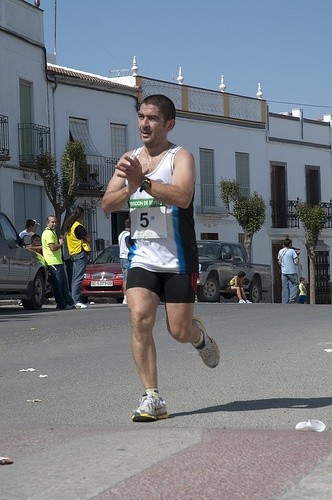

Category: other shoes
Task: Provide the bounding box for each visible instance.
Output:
[56,304,75,310]
[75,302,87,309]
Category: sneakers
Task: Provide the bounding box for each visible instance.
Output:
[239,299,252,304]
[131,394,169,422]
[191,316,220,368]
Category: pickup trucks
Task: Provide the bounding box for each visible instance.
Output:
[196,240,272,303]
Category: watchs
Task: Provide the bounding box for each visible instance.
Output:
[140,177,151,193]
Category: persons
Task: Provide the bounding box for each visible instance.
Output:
[102,94,221,421]
[118,219,132,305]
[17,206,91,309]
[224,271,252,304]
[298,277,309,304]
[278,239,299,304]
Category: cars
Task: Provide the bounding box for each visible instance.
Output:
[78,244,125,304]
[0,211,48,310]
